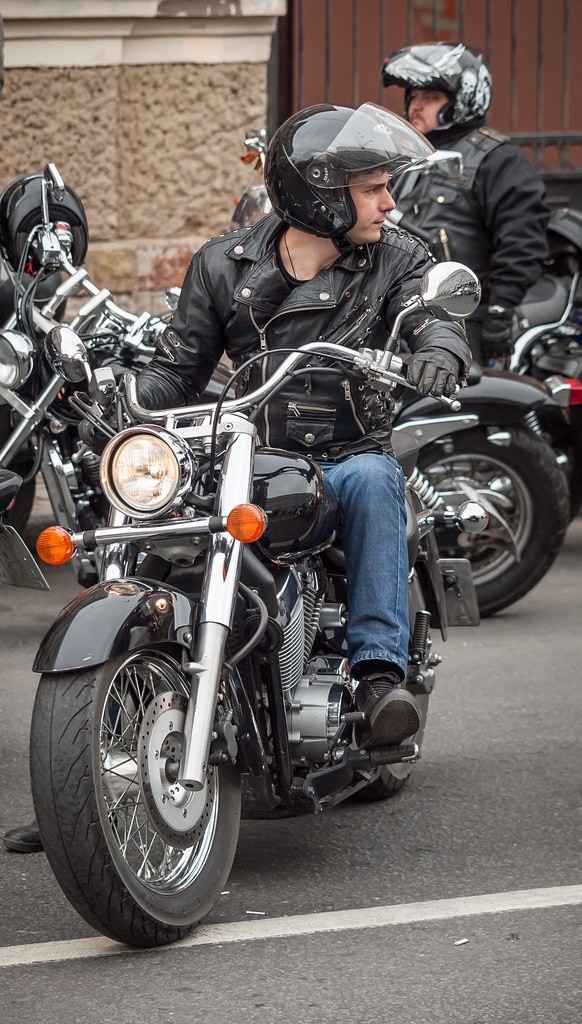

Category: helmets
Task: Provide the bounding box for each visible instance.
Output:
[264,104,413,239]
[0,171,88,273]
[380,41,493,145]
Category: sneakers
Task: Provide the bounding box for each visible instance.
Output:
[355,677,421,751]
[3,808,118,852]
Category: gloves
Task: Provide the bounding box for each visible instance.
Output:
[480,303,515,367]
[406,346,461,396]
[79,401,131,445]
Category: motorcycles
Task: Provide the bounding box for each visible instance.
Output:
[0,126,582,948]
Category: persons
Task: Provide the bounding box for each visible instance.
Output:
[2,102,474,853]
[381,40,553,358]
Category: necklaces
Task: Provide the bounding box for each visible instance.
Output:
[283,229,334,281]
